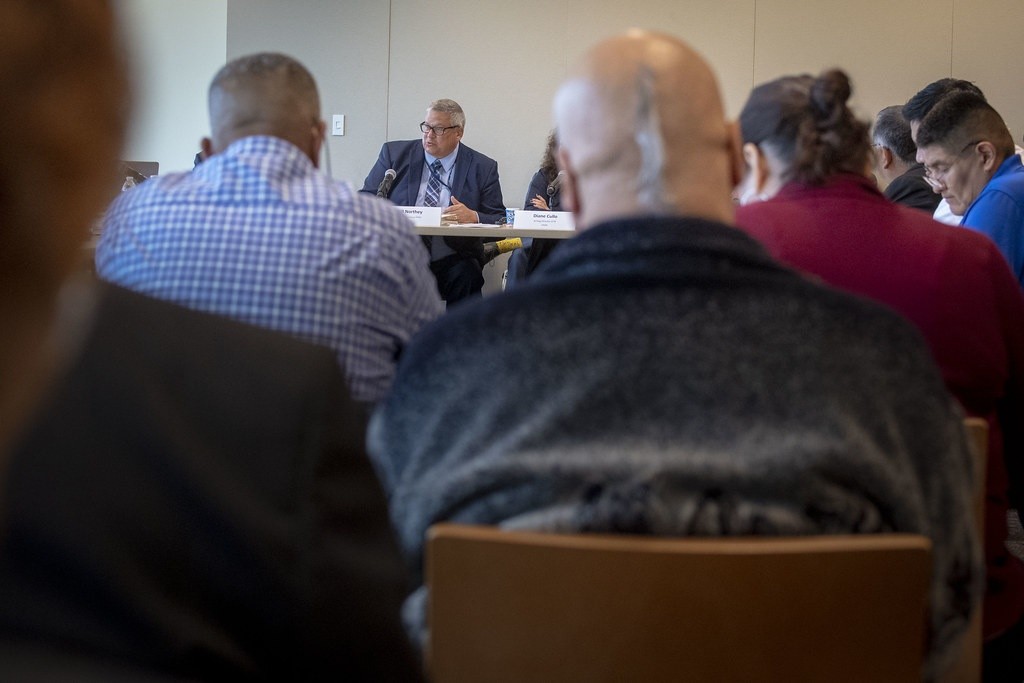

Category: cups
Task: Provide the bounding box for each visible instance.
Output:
[506,207,519,225]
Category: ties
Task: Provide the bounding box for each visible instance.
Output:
[420,160,441,266]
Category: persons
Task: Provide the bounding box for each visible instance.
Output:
[93,51,446,416]
[506,129,565,289]
[1,2,435,682]
[356,98,507,312]
[914,90,1024,285]
[902,75,1024,227]
[367,28,982,660]
[870,104,943,217]
[729,65,1024,681]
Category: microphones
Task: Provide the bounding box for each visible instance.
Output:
[118,161,148,185]
[547,169,568,196]
[376,169,396,198]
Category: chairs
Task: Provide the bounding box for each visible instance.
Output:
[425,524,931,683]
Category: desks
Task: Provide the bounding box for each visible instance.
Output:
[394,205,578,240]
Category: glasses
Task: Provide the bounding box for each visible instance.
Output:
[923,141,977,187]
[420,121,460,136]
